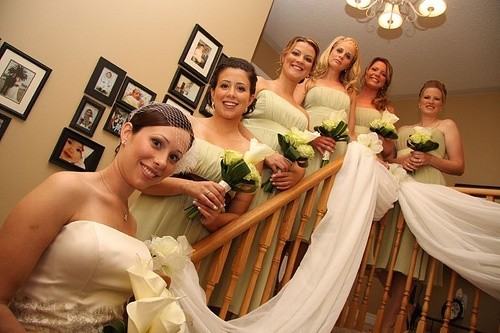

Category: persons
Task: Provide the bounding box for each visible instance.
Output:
[355,57,398,265]
[1,69,22,102]
[198,36,310,317]
[0,102,196,333]
[97,72,112,93]
[191,44,209,67]
[181,81,193,96]
[130,57,263,276]
[124,87,147,109]
[287,36,362,280]
[110,114,125,134]
[79,107,93,128]
[372,81,465,333]
[60,138,94,170]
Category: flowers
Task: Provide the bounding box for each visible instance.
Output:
[314,119,351,168]
[184,137,274,224]
[264,126,320,193]
[369,107,399,140]
[406,126,440,175]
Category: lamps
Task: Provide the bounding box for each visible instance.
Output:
[346,0,447,30]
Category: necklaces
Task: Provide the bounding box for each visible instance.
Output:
[100,171,131,221]
[433,119,439,129]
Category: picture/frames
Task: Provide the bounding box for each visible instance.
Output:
[0,41,53,121]
[209,53,229,85]
[168,66,206,110]
[199,86,215,117]
[178,23,223,84]
[84,56,127,107]
[48,127,105,172]
[162,94,194,119]
[103,102,132,138]
[0,113,12,140]
[116,76,157,111]
[69,95,106,138]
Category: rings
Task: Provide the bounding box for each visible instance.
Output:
[407,163,409,166]
[418,159,420,162]
